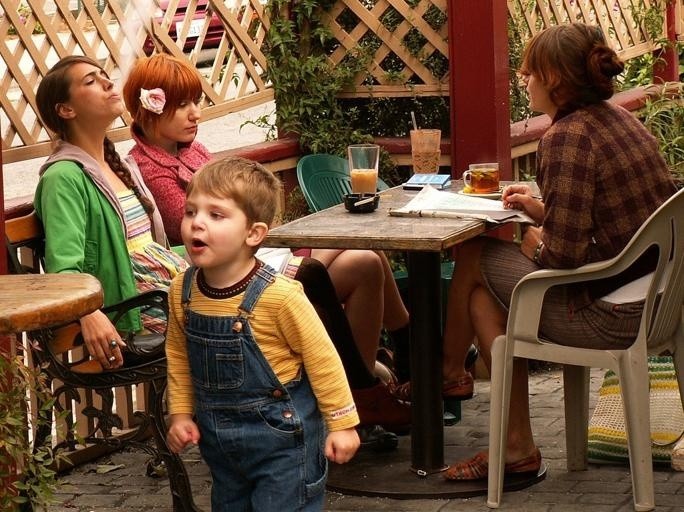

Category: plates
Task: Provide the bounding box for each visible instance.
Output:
[457,186,505,199]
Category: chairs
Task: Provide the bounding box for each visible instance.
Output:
[0,209,202,512]
[295,153,684,511]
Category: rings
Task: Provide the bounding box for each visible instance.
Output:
[108,356,116,361]
[108,338,117,347]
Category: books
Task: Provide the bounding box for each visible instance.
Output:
[386,184,538,227]
[401,172,452,191]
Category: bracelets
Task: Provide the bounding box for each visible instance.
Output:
[533,241,545,263]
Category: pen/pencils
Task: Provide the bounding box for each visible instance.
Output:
[532,196,543,199]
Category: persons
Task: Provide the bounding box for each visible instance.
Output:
[162,154,363,511]
[121,56,481,453]
[33,54,414,438]
[387,23,679,484]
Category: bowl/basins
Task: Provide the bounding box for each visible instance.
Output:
[345,194,378,214]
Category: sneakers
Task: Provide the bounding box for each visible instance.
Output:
[354,316,479,451]
[445,447,541,482]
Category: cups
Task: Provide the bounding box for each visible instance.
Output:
[348,143,380,194]
[409,129,442,176]
[462,162,501,193]
